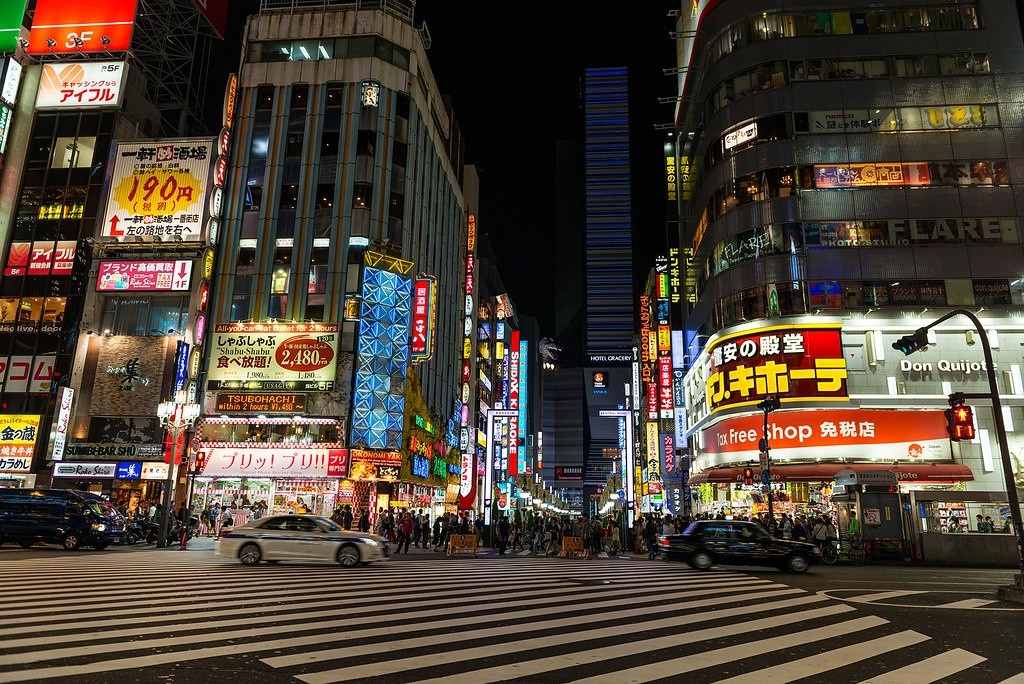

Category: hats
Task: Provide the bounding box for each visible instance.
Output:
[344,505,351,510]
[986,516,991,521]
[1007,516,1012,519]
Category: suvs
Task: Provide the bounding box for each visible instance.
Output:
[0,487,131,552]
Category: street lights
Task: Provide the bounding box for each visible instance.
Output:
[155,390,201,550]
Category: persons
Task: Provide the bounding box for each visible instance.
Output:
[357,508,370,532]
[496,512,621,561]
[949,516,960,533]
[975,514,996,533]
[169,500,233,542]
[631,514,726,561]
[374,507,429,555]
[248,505,264,522]
[732,512,837,563]
[118,499,162,521]
[849,510,860,536]
[1004,516,1013,534]
[431,511,484,552]
[329,504,353,531]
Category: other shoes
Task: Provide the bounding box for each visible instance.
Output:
[434,549,439,552]
[394,551,400,554]
[416,545,421,548]
[423,547,429,549]
[437,545,441,547]
[404,552,407,554]
[443,550,447,552]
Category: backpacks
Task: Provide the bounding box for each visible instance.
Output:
[415,517,421,529]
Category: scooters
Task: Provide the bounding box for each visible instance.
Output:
[123,509,200,547]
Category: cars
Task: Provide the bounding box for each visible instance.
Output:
[657,519,822,575]
[216,513,396,568]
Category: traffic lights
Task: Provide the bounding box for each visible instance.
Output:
[757,399,777,414]
[744,468,753,485]
[892,326,931,356]
[951,405,976,442]
[194,451,206,468]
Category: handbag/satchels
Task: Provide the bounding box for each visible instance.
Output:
[228,518,233,526]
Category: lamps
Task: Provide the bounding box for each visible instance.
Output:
[74,38,90,58]
[87,330,101,335]
[104,329,116,336]
[135,236,144,242]
[151,328,165,337]
[972,306,989,317]
[815,307,826,318]
[172,235,183,242]
[100,35,116,57]
[168,329,181,336]
[19,38,39,61]
[865,306,878,319]
[110,236,119,244]
[674,315,752,376]
[86,238,96,243]
[915,307,935,319]
[47,37,64,62]
[153,234,162,243]
[228,317,324,327]
[219,413,305,420]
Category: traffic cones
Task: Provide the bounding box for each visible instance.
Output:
[177,532,190,551]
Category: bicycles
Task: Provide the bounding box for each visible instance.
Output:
[820,533,867,566]
